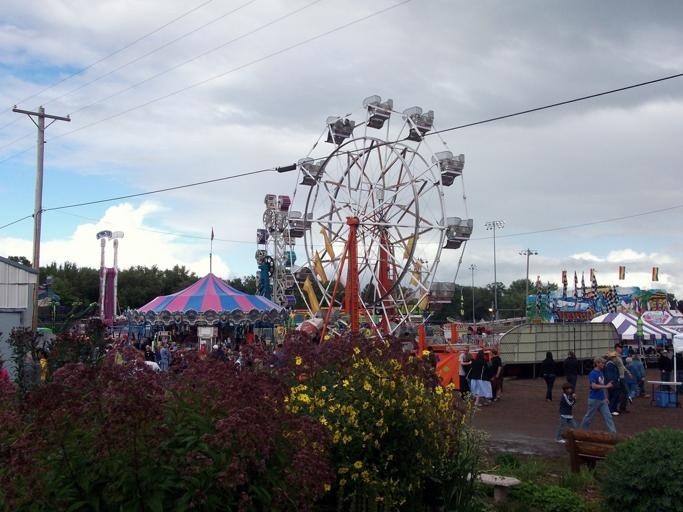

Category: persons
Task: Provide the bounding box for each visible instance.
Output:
[458,342,683,444]
[0,334,283,386]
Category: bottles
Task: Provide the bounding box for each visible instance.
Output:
[636,316,643,336]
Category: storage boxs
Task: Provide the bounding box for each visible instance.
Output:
[653,387,677,408]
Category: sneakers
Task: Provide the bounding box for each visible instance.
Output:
[612,411,619,416]
[555,438,565,443]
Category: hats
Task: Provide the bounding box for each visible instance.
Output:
[594,358,606,363]
[608,352,617,357]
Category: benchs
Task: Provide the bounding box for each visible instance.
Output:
[558,425,636,479]
